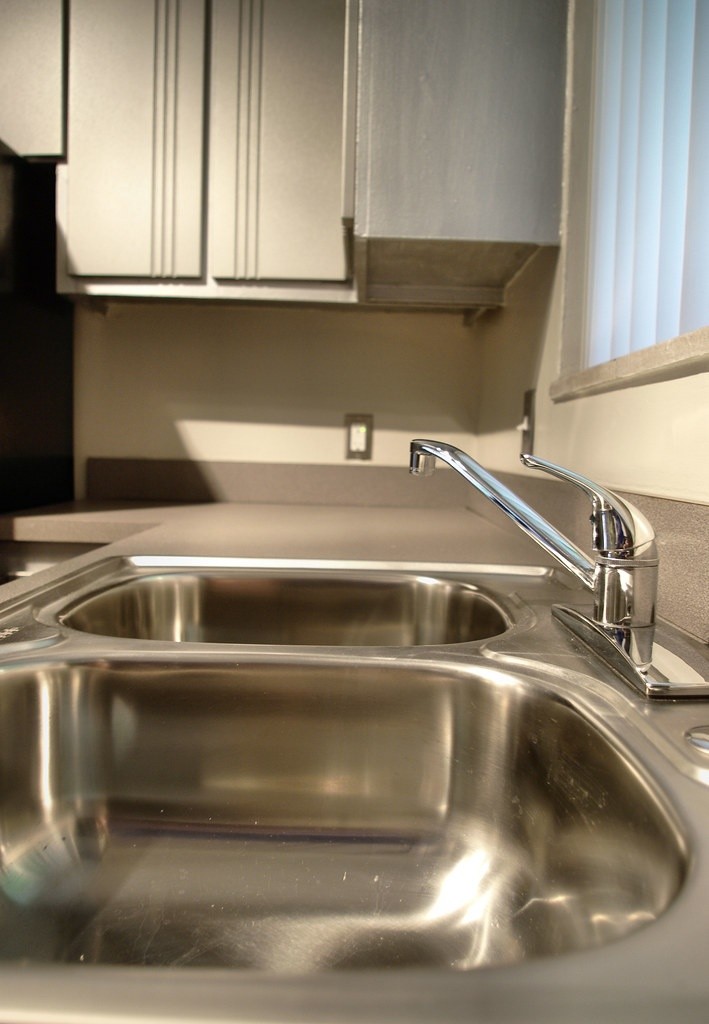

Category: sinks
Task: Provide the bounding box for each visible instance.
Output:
[0,653,709,1022]
[20,552,603,650]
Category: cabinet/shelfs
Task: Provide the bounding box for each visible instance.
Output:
[0,0,565,515]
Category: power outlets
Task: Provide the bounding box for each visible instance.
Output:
[344,413,375,458]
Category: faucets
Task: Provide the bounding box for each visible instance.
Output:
[406,436,654,627]
